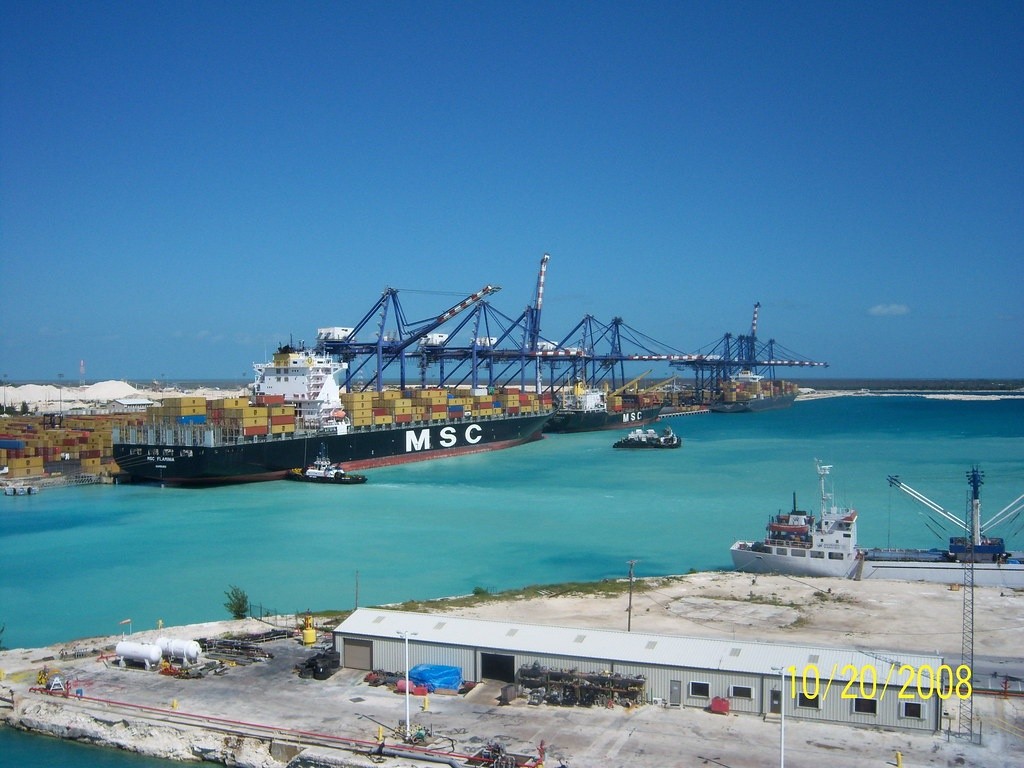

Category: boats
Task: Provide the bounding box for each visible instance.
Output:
[542,377,667,434]
[287,441,368,485]
[710,371,800,413]
[612,428,682,450]
[728,456,1024,586]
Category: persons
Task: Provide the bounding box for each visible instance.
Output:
[306,608,312,615]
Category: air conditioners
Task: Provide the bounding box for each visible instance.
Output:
[652,698,662,705]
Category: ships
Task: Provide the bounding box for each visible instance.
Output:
[112,332,565,484]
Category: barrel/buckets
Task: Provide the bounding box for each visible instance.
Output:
[76,689,82,698]
